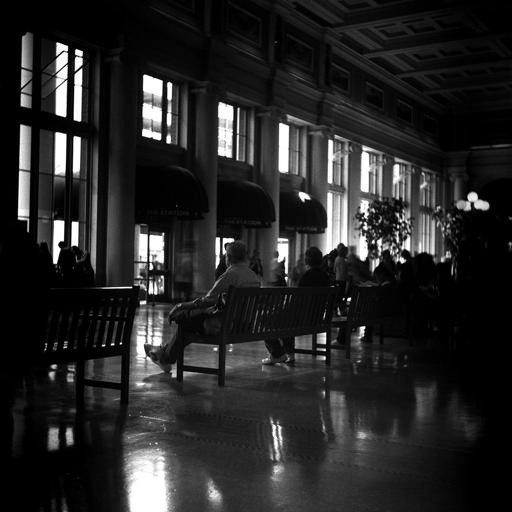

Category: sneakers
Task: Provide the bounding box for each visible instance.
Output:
[261,354,288,365]
[285,353,296,363]
[149,345,176,365]
[144,343,173,373]
[331,339,347,347]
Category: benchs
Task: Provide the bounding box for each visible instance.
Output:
[312,286,414,359]
[177,285,340,386]
[35,285,140,405]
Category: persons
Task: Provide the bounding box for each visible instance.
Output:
[143,241,263,373]
[2,225,94,390]
[262,242,369,365]
[361,225,510,359]
